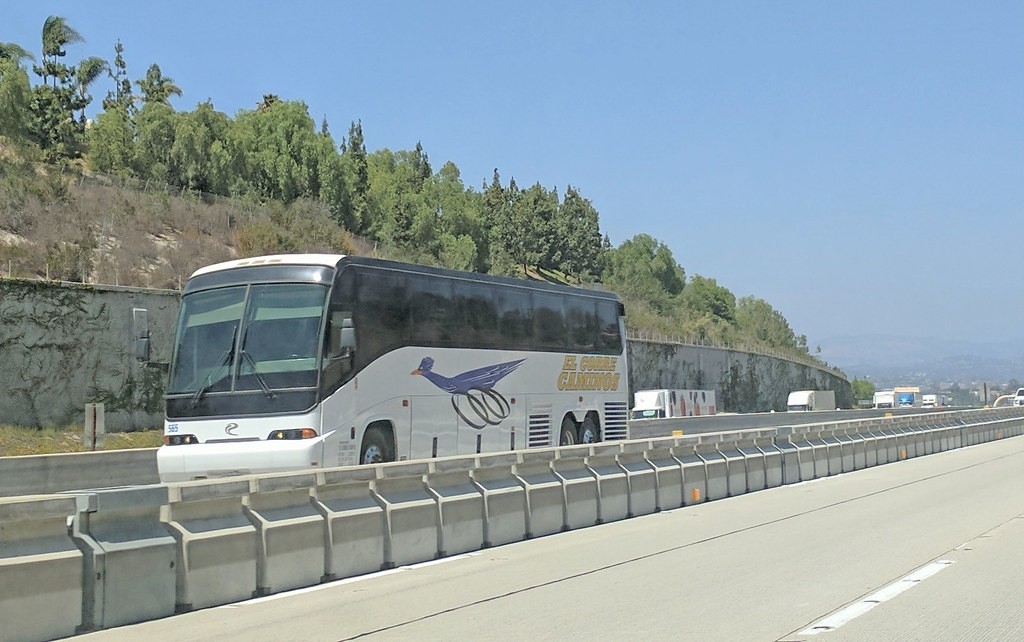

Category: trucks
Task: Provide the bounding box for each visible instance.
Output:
[787,389,836,411]
[632,390,717,419]
[873,387,942,410]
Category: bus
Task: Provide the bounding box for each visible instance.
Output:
[135,253,632,487]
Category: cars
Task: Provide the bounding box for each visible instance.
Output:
[1012,388,1023,407]
